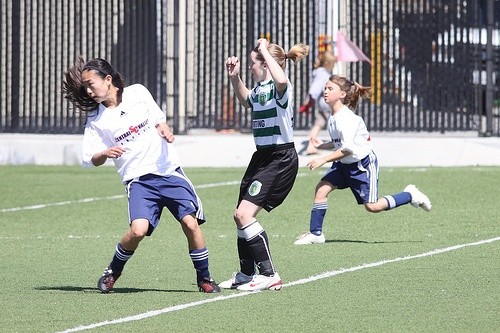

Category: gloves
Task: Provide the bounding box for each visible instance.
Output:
[299,98,315,114]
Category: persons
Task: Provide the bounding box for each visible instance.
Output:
[217,38,309,291]
[299,52,337,154]
[60,56,221,293]
[294,75,431,245]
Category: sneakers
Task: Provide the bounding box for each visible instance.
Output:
[218,269,283,291]
[97,267,121,293]
[403,184,432,211]
[197,277,222,293]
[293,231,325,245]
